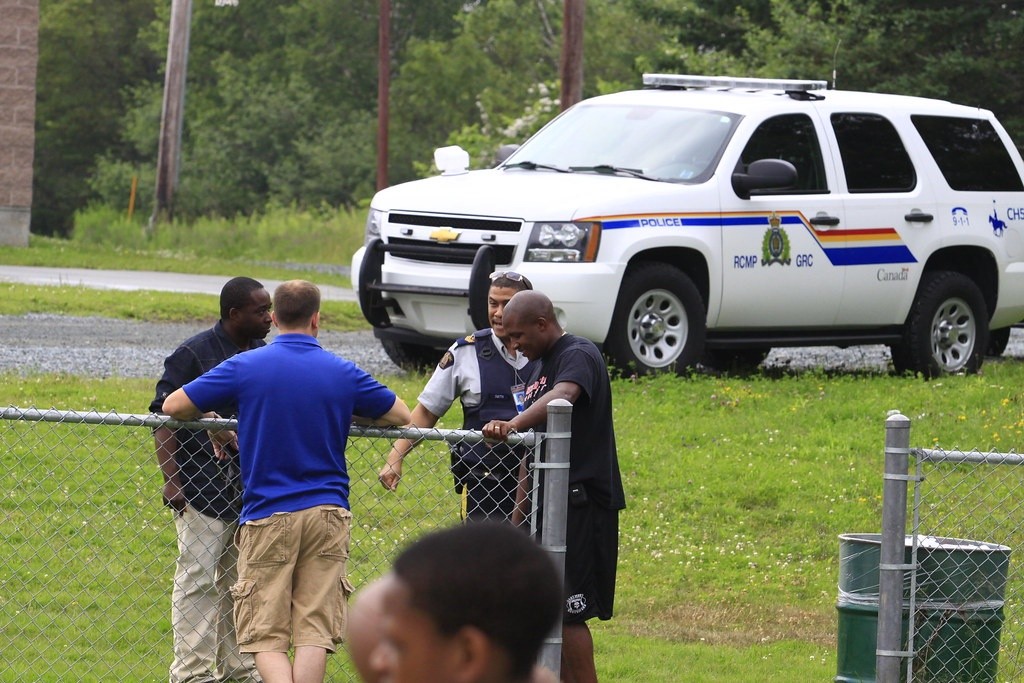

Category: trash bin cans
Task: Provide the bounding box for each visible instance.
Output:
[826,531,1012,683]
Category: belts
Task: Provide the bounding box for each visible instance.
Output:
[473,469,518,483]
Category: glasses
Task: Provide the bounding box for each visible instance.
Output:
[489,271,530,289]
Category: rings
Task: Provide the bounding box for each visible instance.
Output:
[494,426,500,429]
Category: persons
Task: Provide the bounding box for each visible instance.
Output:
[160,278,412,682]
[481,288,628,683]
[146,276,277,682]
[376,271,545,523]
[517,394,524,404]
[367,516,565,682]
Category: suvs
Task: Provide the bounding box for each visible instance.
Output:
[349,71,1024,384]
